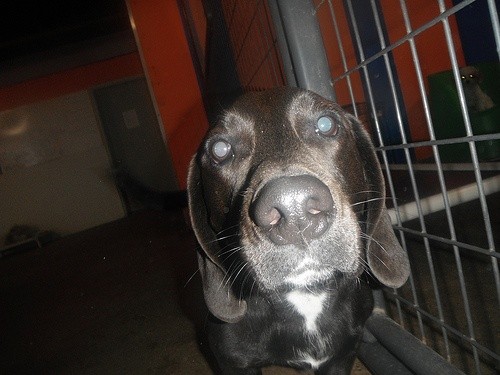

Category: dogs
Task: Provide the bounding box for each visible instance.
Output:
[120,85,411,375]
[451,66,494,114]
